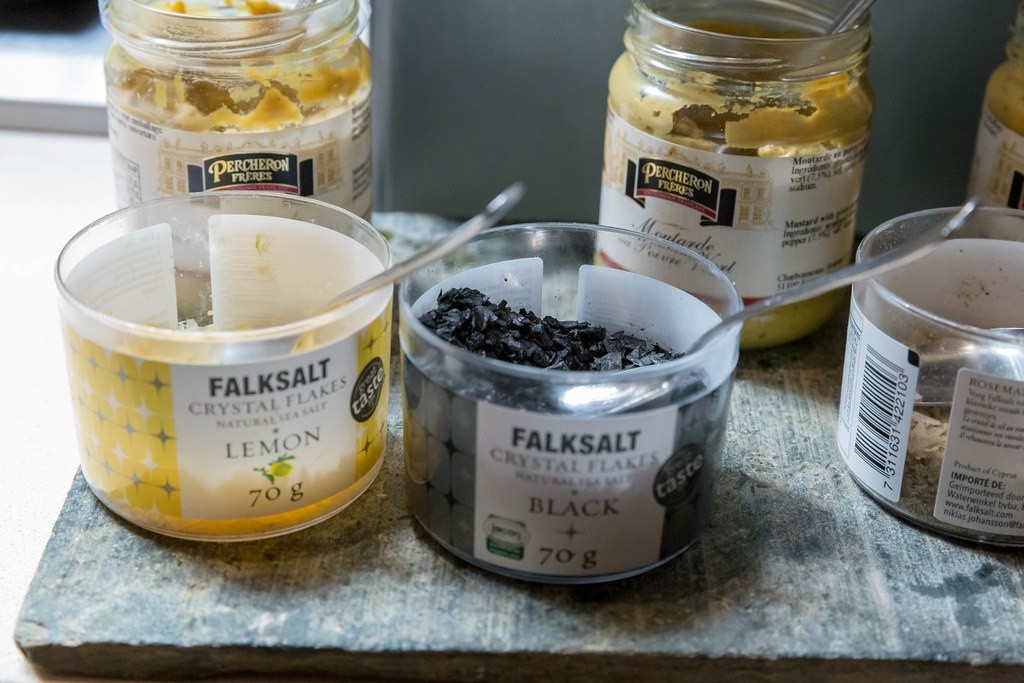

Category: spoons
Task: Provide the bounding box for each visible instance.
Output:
[552,196,982,421]
[911,322,1024,404]
